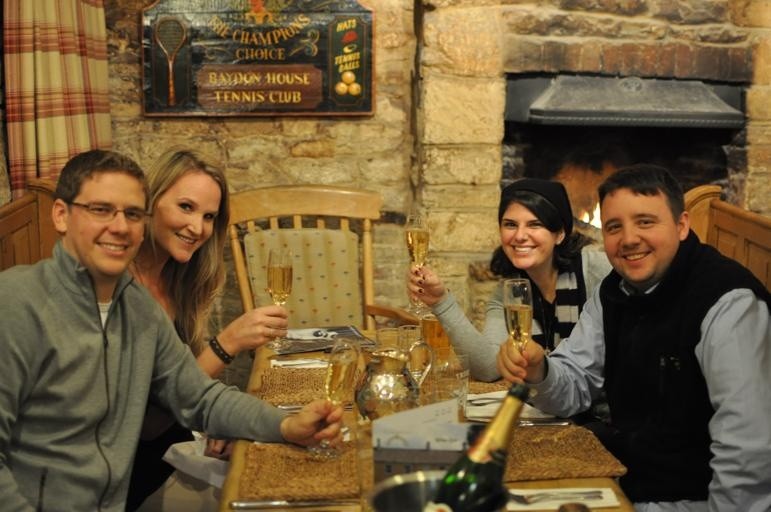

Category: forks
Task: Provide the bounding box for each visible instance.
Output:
[508,490,604,505]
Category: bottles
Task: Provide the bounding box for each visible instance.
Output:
[423,381,532,512]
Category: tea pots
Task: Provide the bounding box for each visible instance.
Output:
[355,339,433,423]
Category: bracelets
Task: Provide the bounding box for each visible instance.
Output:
[208,336,234,364]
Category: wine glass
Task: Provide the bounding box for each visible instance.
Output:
[403,214,430,313]
[503,277,533,354]
[307,337,362,457]
[265,248,293,352]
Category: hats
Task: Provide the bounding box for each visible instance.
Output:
[500,178,572,234]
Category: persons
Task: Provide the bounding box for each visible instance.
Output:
[405,179,613,428]
[1,148,345,511]
[126,144,290,502]
[495,164,771,512]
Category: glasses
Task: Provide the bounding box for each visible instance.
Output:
[63,200,152,222]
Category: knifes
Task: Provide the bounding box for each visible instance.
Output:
[229,498,362,510]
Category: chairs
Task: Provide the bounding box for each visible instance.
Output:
[1,176,57,271]
[682,184,722,242]
[219,185,384,344]
[707,198,771,291]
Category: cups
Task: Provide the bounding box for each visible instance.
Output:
[431,346,469,422]
[421,314,451,371]
[376,328,398,350]
[398,324,424,383]
